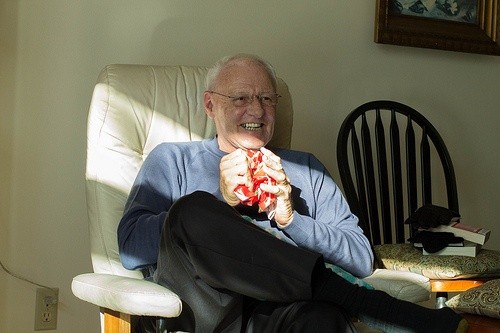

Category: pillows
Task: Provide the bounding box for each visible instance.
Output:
[448,279,500,317]
[374,240,499,280]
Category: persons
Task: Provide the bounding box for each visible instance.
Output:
[117,54,468,333]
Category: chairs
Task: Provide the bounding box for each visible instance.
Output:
[335,100,499,309]
[72,63,430,332]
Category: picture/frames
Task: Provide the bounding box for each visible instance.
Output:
[373,0,500,57]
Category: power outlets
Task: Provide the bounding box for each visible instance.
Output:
[36,288,58,330]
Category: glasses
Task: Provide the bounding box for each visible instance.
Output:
[205,91,282,106]
